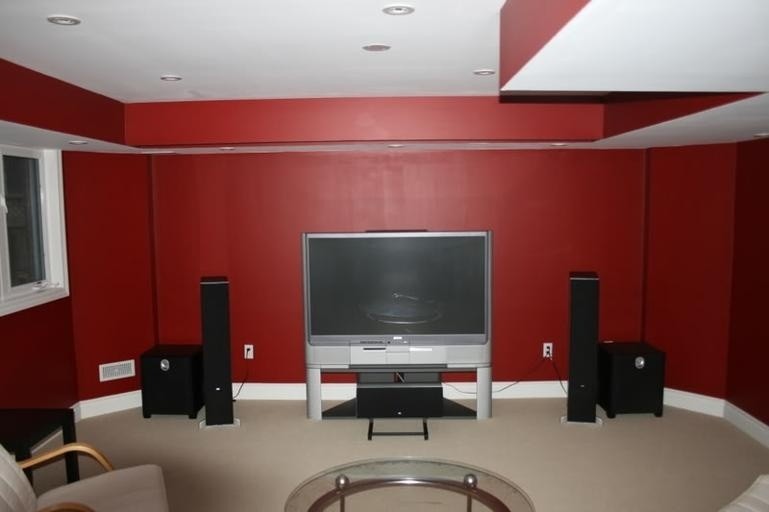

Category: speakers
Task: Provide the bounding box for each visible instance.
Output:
[201,276,235,425]
[568,272,601,422]
[600,341,664,420]
[356,383,444,419]
[140,345,201,419]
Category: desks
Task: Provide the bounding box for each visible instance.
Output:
[0,407,80,498]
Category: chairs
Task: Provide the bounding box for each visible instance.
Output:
[0,441,175,512]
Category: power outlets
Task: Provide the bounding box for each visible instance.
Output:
[243,344,255,360]
[542,342,553,358]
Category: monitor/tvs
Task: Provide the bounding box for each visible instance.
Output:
[306,230,489,348]
